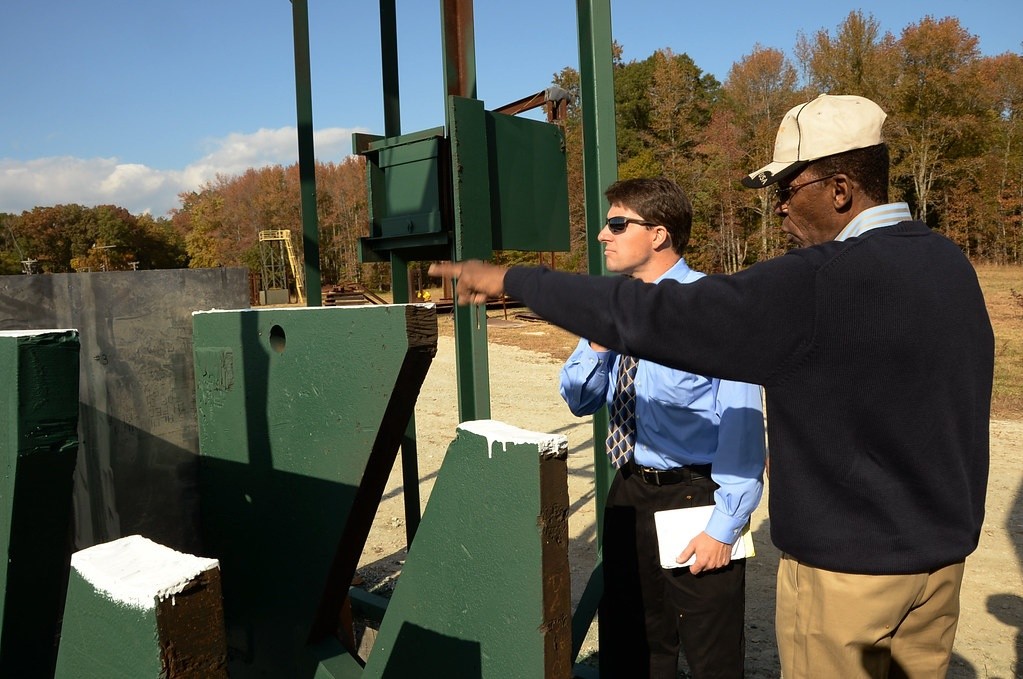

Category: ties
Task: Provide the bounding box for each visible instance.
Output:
[604,354,638,470]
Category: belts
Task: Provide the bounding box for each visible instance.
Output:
[628,451,710,486]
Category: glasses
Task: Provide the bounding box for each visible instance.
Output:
[606,215,659,232]
[774,172,853,207]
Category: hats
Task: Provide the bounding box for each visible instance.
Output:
[740,93,887,188]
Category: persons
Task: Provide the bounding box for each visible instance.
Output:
[428,93,994,678]
[558,175,766,679]
